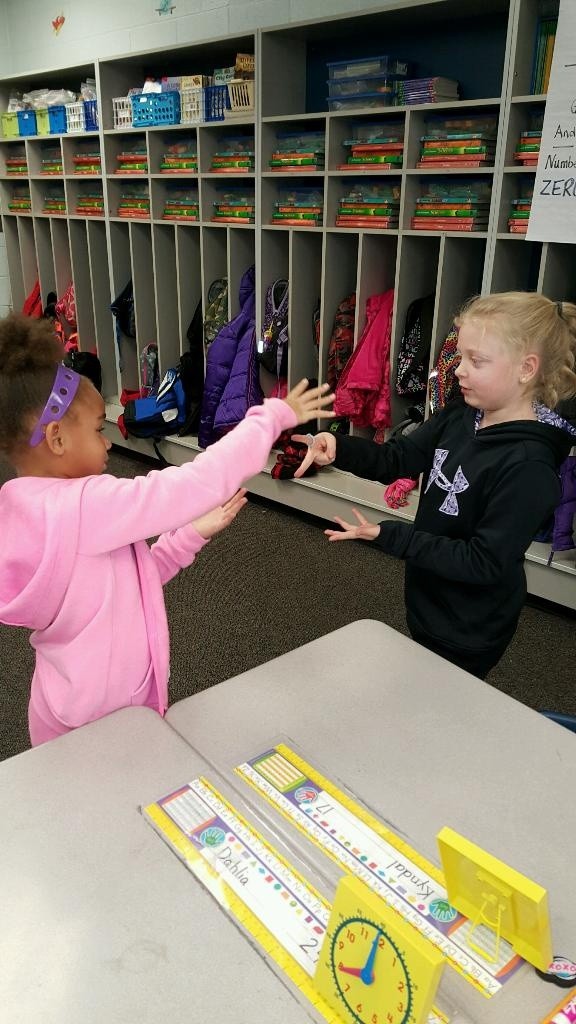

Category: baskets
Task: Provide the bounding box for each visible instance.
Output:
[179,88,204,123]
[225,80,255,111]
[112,96,132,130]
[152,92,179,126]
[204,86,230,121]
[2,99,98,138]
[132,92,153,127]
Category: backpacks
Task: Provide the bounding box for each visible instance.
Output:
[123,368,187,439]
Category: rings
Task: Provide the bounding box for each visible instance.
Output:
[307,434,314,447]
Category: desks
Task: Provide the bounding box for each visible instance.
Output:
[0,617,576,1024]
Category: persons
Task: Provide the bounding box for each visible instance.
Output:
[1,315,337,747]
[290,291,576,680]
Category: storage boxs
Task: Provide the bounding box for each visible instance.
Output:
[0,74,255,138]
[326,54,411,114]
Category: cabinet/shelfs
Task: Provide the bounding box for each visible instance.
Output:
[0,0,576,611]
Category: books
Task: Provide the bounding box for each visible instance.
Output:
[8,194,104,216]
[162,199,198,220]
[534,22,557,94]
[513,131,542,166]
[415,134,495,167]
[268,149,324,171]
[335,138,403,170]
[336,199,400,229]
[208,152,255,173]
[398,77,459,105]
[117,195,150,218]
[5,153,101,175]
[210,199,255,223]
[411,183,490,232]
[159,153,198,173]
[271,202,324,226]
[114,151,147,174]
[507,192,532,234]
